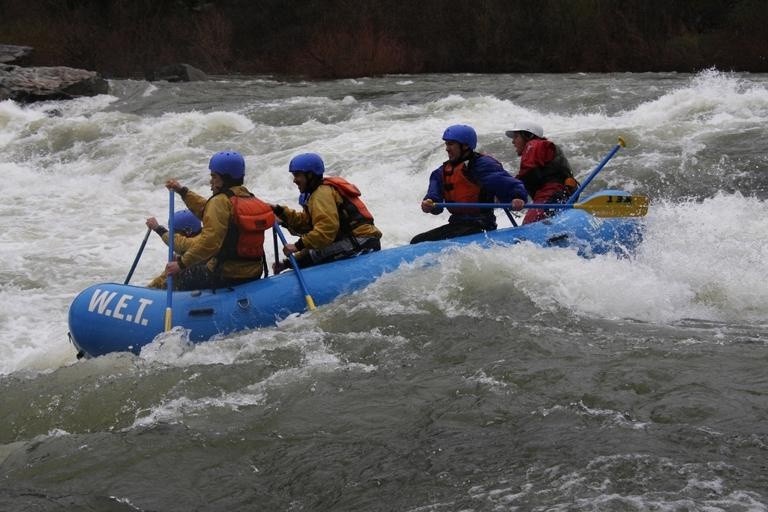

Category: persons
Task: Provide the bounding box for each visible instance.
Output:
[505,121,579,227]
[410,124,527,244]
[145,209,203,291]
[165,151,274,293]
[267,153,380,276]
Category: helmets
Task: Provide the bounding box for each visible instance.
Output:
[289,153,324,175]
[443,125,476,149]
[506,120,543,139]
[209,151,244,180]
[168,210,201,236]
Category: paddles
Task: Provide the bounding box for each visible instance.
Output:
[431,195,649,218]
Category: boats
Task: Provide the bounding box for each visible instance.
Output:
[65,189,648,366]
